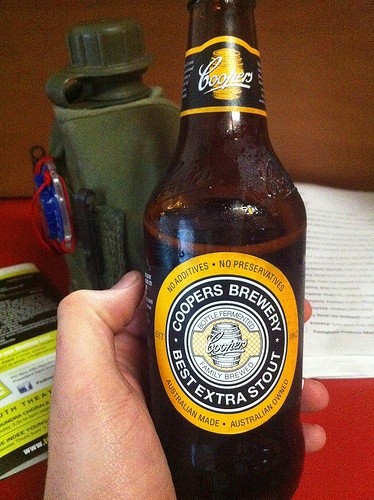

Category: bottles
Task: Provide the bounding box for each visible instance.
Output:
[41,19,183,293]
[140,0,308,500]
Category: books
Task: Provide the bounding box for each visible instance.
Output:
[0,263,61,480]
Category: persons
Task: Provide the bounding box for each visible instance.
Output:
[44,270,330,500]
